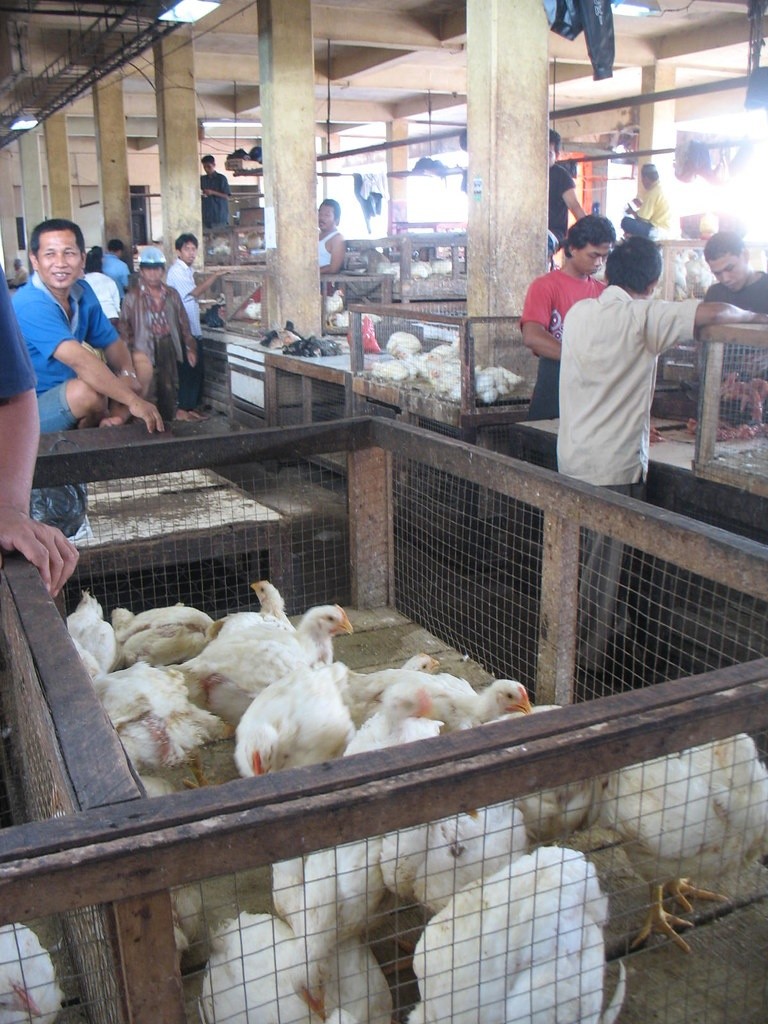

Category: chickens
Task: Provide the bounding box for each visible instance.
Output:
[405,846,625,1024]
[377,259,453,280]
[325,289,344,327]
[334,310,382,327]
[198,907,394,1024]
[232,661,358,778]
[603,733,768,953]
[349,652,604,911]
[49,579,353,956]
[273,835,387,1024]
[372,332,524,403]
[0,921,63,1024]
[244,299,262,322]
[651,247,720,300]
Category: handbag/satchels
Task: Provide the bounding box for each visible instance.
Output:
[346,316,382,354]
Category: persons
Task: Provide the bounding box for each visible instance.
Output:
[620,163,670,240]
[521,216,616,422]
[10,219,231,433]
[0,265,79,599]
[200,156,232,228]
[317,199,347,274]
[9,259,28,288]
[556,237,768,681]
[704,232,768,315]
[547,129,588,255]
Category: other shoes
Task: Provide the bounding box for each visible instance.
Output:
[575,663,642,690]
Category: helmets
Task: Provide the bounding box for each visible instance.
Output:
[138,246,167,265]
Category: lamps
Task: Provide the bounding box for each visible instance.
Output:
[10,115,39,130]
[158,0,221,23]
